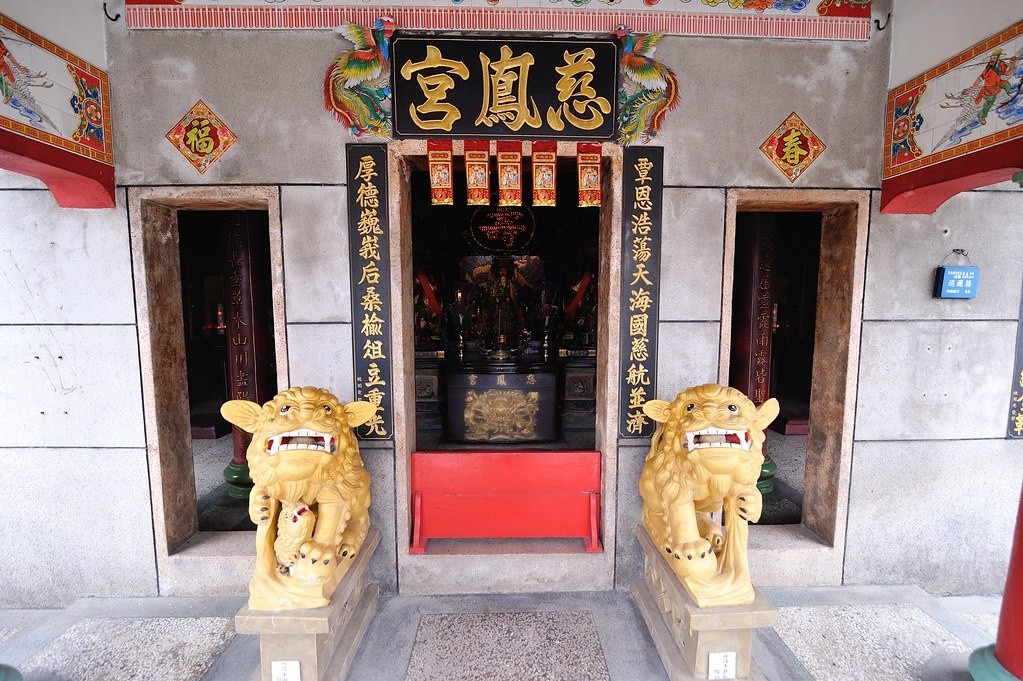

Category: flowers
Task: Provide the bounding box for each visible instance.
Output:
[534,303,564,330]
